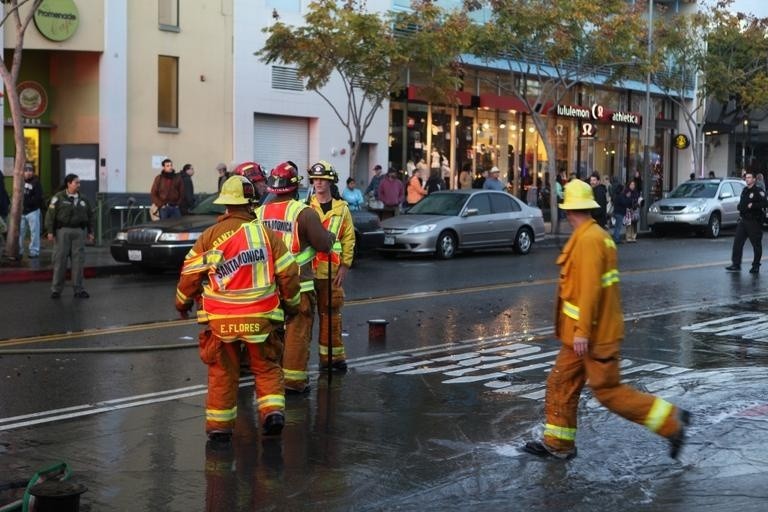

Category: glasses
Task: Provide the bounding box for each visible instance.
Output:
[23,166,33,172]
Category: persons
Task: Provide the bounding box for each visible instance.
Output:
[175,174,302,450]
[216,163,232,192]
[149,159,185,222]
[521,164,662,244]
[0,170,10,241]
[216,162,268,376]
[341,160,510,222]
[178,165,197,215]
[253,162,336,394]
[689,168,766,190]
[724,171,768,273]
[44,175,96,299]
[300,159,356,374]
[19,161,43,259]
[524,179,692,461]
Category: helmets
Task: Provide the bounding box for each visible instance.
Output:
[308,160,339,182]
[558,178,602,211]
[235,162,268,184]
[212,175,260,205]
[266,161,299,194]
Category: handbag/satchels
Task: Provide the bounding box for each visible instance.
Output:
[606,201,615,216]
[149,203,161,221]
[622,208,632,227]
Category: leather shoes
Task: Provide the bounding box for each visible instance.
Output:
[50,291,61,298]
[750,266,759,273]
[669,409,691,460]
[726,265,740,270]
[211,432,232,449]
[262,414,285,436]
[75,291,90,298]
[331,359,347,370]
[524,441,577,459]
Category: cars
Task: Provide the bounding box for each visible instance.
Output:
[648,170,759,238]
[107,187,381,272]
[379,184,546,258]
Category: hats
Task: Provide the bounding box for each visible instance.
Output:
[490,166,500,173]
[372,164,382,171]
[216,163,226,171]
